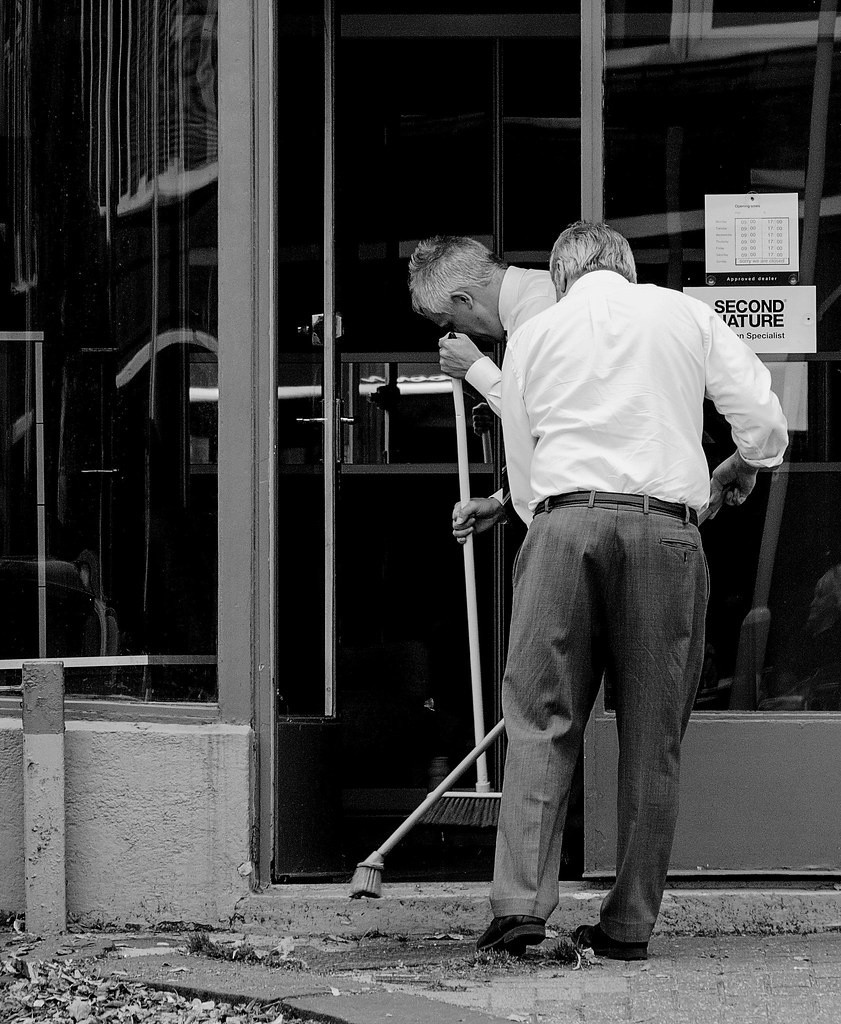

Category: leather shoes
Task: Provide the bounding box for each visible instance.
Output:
[572,923,648,960]
[477,916,546,958]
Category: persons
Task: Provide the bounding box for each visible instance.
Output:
[471,223,792,959]
[408,235,563,545]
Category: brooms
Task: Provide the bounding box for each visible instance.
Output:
[413,333,500,828]
[352,485,726,898]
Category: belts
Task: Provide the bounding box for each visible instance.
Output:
[533,490,699,528]
